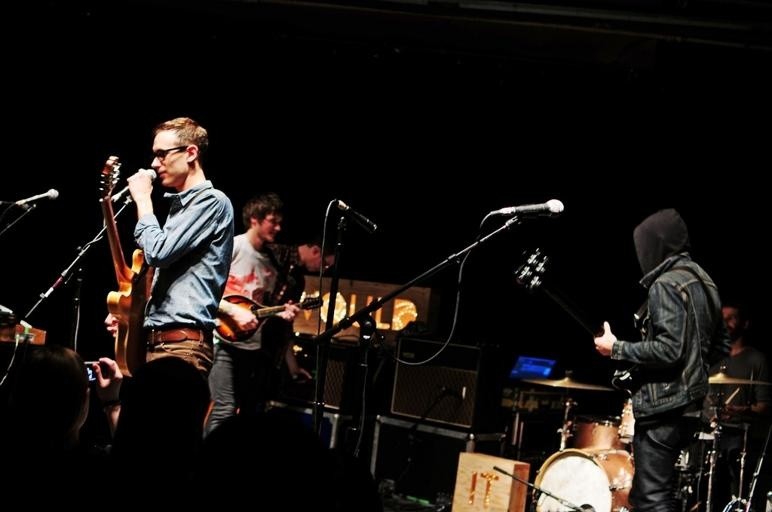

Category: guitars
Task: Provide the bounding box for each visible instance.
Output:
[100,157,152,377]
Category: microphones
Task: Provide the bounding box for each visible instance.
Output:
[493,199,565,218]
[110,169,157,203]
[335,200,377,233]
[16,188,59,208]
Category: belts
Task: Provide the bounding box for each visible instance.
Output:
[142,326,212,345]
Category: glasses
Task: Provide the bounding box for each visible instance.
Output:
[152,146,190,161]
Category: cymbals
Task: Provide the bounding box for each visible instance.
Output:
[707,373,765,385]
[520,378,612,392]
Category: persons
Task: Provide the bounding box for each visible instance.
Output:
[206,193,298,433]
[264,237,336,380]
[127,116,235,378]
[718,301,767,450]
[592,208,731,512]
[0,346,385,512]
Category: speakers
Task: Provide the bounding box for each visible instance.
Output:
[292,340,364,414]
[391,337,486,429]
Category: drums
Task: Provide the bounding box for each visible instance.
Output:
[618,398,637,444]
[532,449,637,511]
[571,416,631,451]
